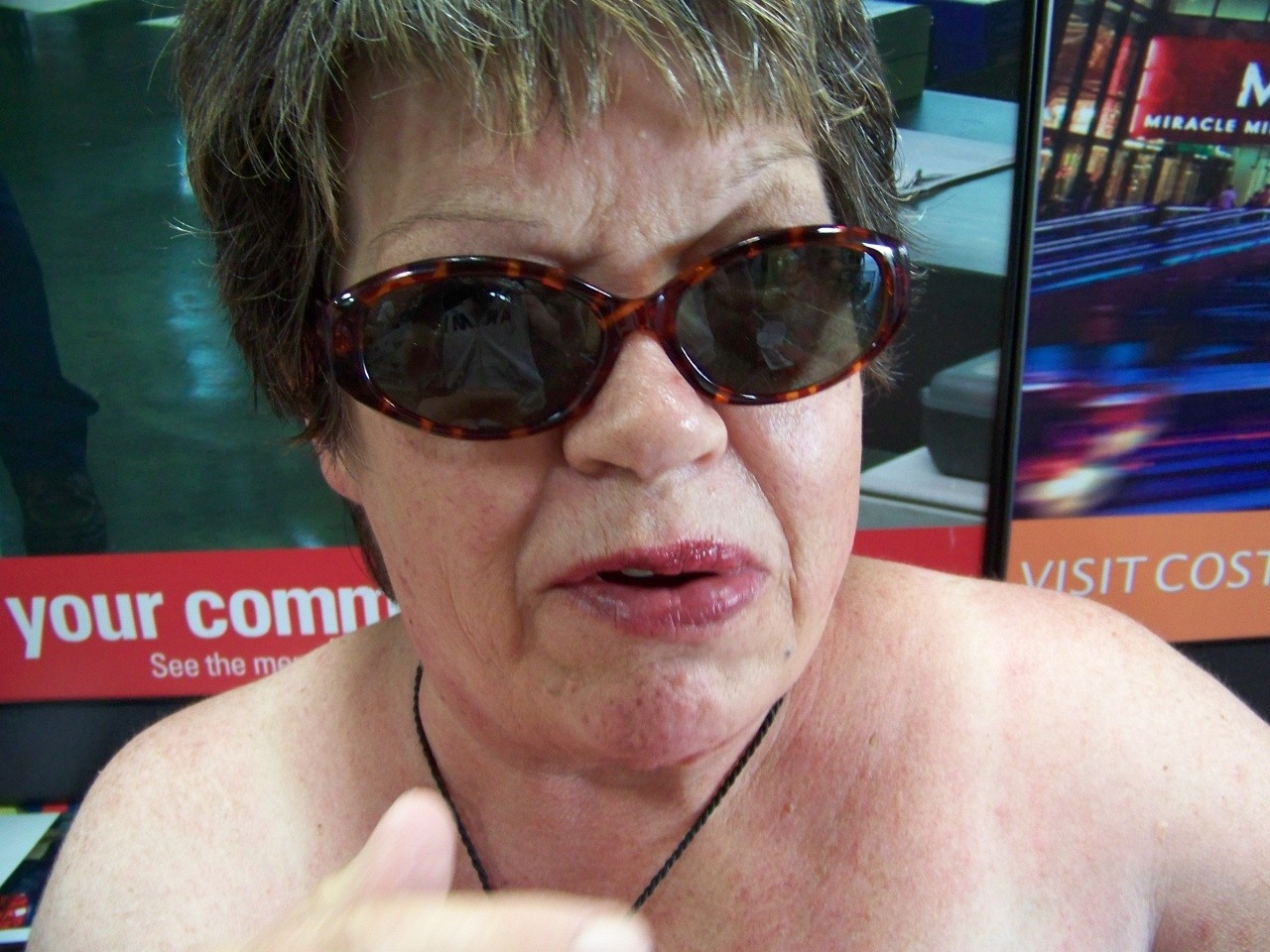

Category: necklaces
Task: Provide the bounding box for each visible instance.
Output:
[412,660,787,917]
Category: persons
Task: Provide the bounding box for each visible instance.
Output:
[22,1,1269,951]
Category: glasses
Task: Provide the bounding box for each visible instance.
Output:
[307,227,911,443]
